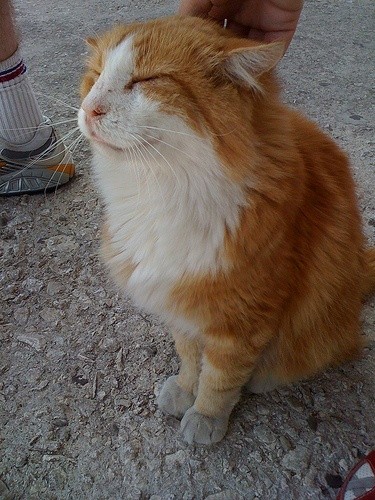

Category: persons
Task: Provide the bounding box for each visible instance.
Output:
[1,0,305,196]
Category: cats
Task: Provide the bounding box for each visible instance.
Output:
[0,17,374,447]
